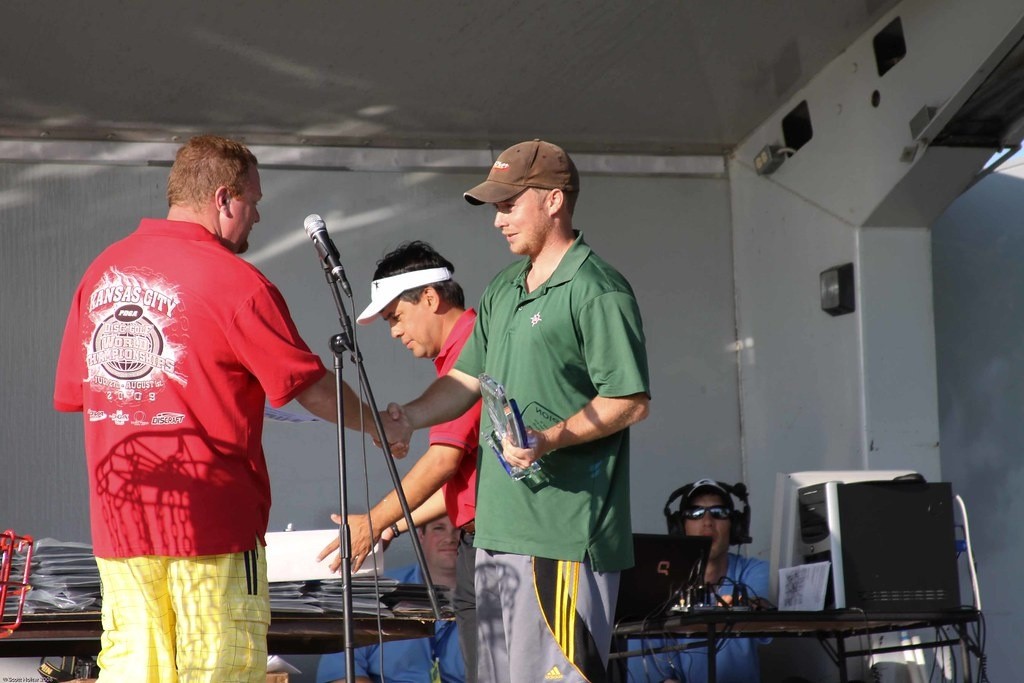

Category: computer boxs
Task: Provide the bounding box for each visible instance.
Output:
[797,481,961,613]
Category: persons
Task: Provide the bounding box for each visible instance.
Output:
[317,513,468,683]
[317,240,489,683]
[53,135,414,683]
[627,479,777,683]
[375,139,652,683]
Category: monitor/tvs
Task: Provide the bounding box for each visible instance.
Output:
[767,469,918,609]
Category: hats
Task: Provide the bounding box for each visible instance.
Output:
[462,138,581,206]
[356,266,453,327]
[679,478,735,512]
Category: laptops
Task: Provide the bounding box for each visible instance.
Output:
[612,533,712,626]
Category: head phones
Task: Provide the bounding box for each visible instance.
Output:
[664,480,753,545]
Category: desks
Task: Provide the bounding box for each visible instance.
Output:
[0,613,455,683]
[609,618,972,683]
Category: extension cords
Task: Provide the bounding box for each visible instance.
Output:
[688,604,753,612]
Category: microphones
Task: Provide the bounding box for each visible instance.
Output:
[303,214,353,297]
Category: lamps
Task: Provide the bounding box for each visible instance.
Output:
[754,144,797,177]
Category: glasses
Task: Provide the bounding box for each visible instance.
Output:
[681,504,732,520]
